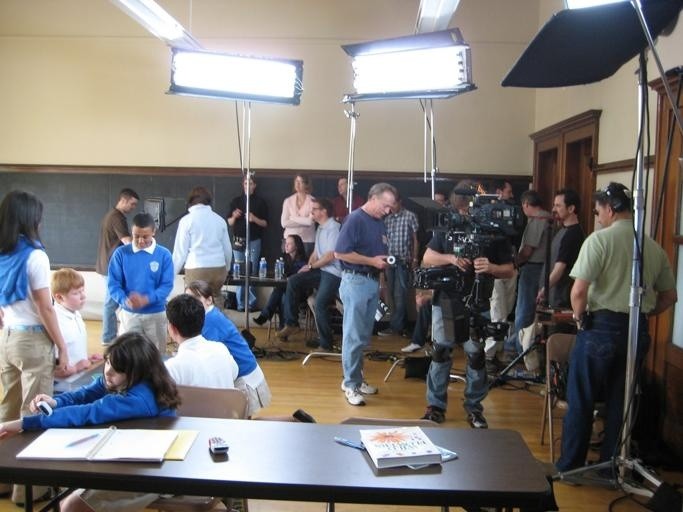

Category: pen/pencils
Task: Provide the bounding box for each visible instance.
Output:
[68,434,98,447]
[236,209,247,215]
[333,437,365,450]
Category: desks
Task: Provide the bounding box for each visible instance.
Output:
[0,411,554,511]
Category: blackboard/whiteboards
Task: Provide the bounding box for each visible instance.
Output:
[0,163,533,272]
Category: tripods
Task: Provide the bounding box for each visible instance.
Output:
[547,48,683,499]
[302,103,364,378]
[486,220,553,446]
[383,99,469,383]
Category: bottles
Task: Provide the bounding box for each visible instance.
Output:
[233,258,240,280]
[259,257,267,278]
[279,256,284,277]
[275,259,280,281]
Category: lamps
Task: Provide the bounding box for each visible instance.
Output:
[113,0,681,512]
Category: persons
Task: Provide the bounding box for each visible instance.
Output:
[0,332,182,512]
[162,293,239,389]
[534,181,679,487]
[107,213,174,355]
[401,179,585,405]
[50,267,103,378]
[227,174,269,312]
[421,198,512,429]
[95,188,140,345]
[185,280,317,423]
[251,171,421,352]
[0,190,68,506]
[334,183,399,405]
[171,184,233,314]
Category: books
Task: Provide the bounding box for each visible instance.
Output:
[15,425,179,463]
[407,444,457,470]
[360,425,442,470]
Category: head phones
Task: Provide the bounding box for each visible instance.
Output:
[604,185,625,212]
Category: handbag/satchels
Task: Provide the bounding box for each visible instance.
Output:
[517,324,547,371]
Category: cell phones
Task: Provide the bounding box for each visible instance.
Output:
[36,401,52,416]
[209,437,228,453]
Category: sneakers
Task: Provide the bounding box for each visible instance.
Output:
[275,325,300,337]
[420,405,446,423]
[401,341,425,352]
[341,377,379,394]
[378,327,402,336]
[345,389,366,406]
[467,411,488,429]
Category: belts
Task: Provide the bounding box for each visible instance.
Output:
[344,268,376,278]
